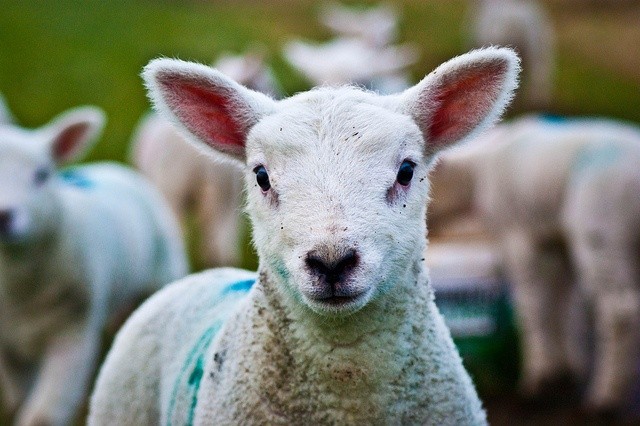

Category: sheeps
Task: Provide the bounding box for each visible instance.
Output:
[125,48,280,270]
[281,36,420,92]
[426,112,640,418]
[85,41,525,425]
[0,96,188,426]
